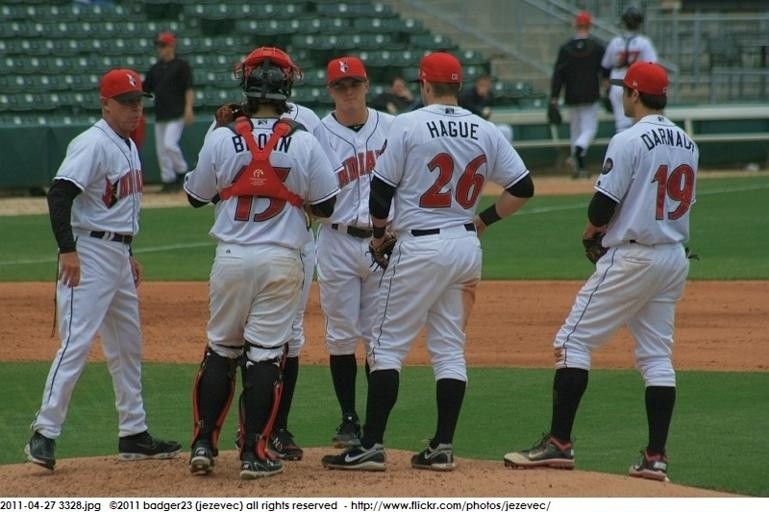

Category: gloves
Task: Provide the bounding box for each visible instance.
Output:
[603,95,614,113]
[548,104,562,125]
[583,235,608,264]
[369,235,397,269]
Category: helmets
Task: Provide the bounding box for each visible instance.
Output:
[247,66,289,100]
[621,7,644,29]
[242,46,300,79]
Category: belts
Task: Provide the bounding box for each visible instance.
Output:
[411,223,476,236]
[332,222,373,238]
[90,230,133,244]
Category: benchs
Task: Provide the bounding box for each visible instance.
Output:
[484,104,769,149]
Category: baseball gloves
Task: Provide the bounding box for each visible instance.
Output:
[582,232,609,266]
[365,236,395,270]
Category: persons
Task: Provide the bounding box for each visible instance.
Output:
[459,76,495,116]
[25,67,178,468]
[602,4,659,136]
[323,53,535,470]
[315,56,396,443]
[550,10,609,177]
[185,49,341,475]
[379,72,418,114]
[507,60,700,484]
[141,31,192,191]
[208,45,322,457]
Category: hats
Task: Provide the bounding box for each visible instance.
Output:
[328,57,367,87]
[575,12,591,27]
[153,33,175,44]
[101,68,153,102]
[609,61,671,96]
[408,52,461,84]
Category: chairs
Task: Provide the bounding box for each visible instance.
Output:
[0,0,550,130]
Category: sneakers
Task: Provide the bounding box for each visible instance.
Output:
[629,449,668,480]
[119,431,183,461]
[189,444,214,476]
[410,440,455,471]
[567,156,579,178]
[240,458,284,479]
[321,444,389,473]
[503,435,576,469]
[24,432,56,471]
[331,414,361,448]
[267,427,304,462]
